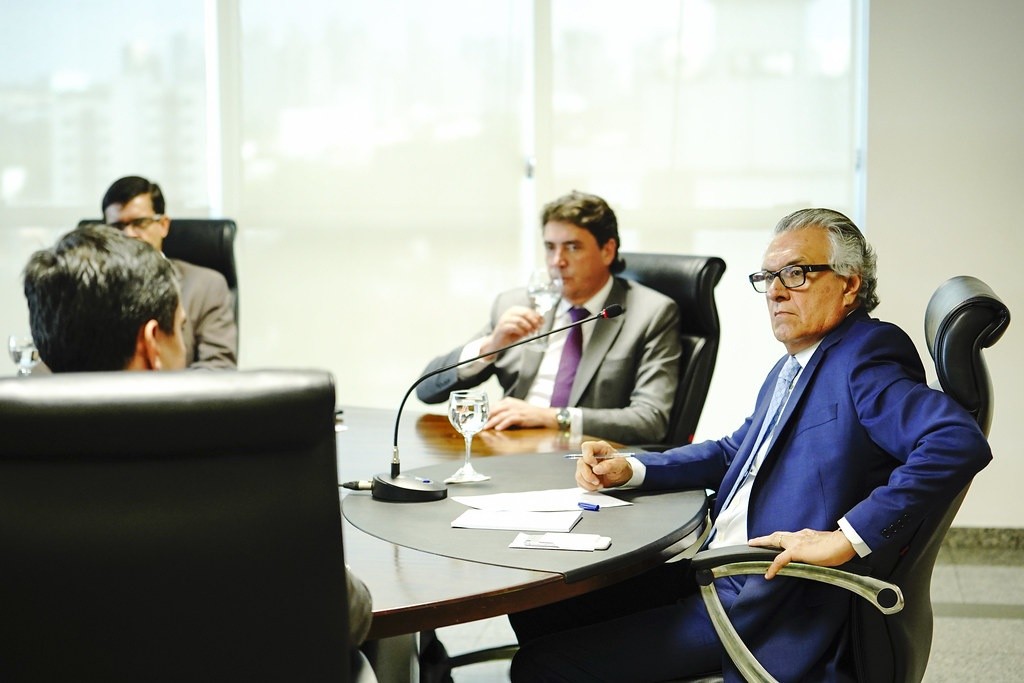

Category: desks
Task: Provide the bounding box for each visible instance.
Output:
[332,405,708,683]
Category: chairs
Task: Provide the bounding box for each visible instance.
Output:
[77,220,239,368]
[617,252,728,451]
[0,368,379,683]
[689,275,1011,683]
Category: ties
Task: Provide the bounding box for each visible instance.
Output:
[700,354,802,553]
[550,305,591,407]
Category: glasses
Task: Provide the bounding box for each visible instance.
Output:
[748,264,848,293]
[107,214,162,231]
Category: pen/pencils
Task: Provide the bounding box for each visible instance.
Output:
[564,452,636,459]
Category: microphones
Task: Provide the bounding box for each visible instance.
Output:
[375,305,623,504]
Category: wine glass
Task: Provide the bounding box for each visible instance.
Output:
[447,390,491,483]
[513,265,564,352]
[8,335,42,376]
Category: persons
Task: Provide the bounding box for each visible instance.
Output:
[22,225,419,682]
[511,210,992,683]
[102,176,237,368]
[417,190,681,447]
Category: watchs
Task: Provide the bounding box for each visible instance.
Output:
[557,408,569,430]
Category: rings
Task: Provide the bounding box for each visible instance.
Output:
[779,535,783,545]
